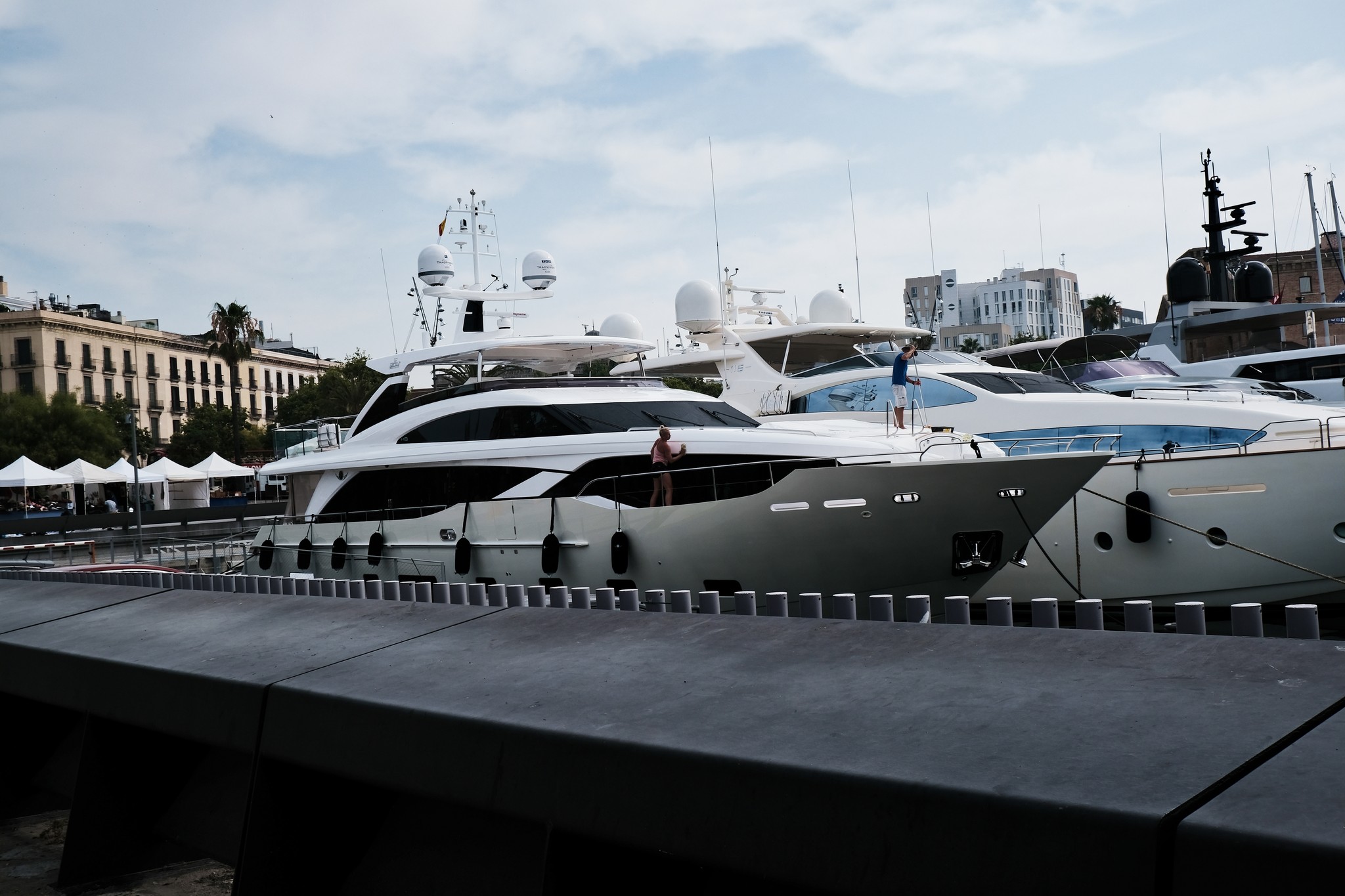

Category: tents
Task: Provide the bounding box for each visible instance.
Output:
[0,452,255,518]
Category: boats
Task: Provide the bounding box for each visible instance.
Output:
[236,184,1345,611]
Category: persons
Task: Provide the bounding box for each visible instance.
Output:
[649,428,686,507]
[104,497,117,514]
[231,454,280,465]
[891,344,921,429]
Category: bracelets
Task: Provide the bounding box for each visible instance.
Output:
[912,381,915,385]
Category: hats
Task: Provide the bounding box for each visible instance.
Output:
[129,508,134,512]
[36,505,42,508]
[29,505,35,509]
[902,344,918,356]
[24,505,30,510]
